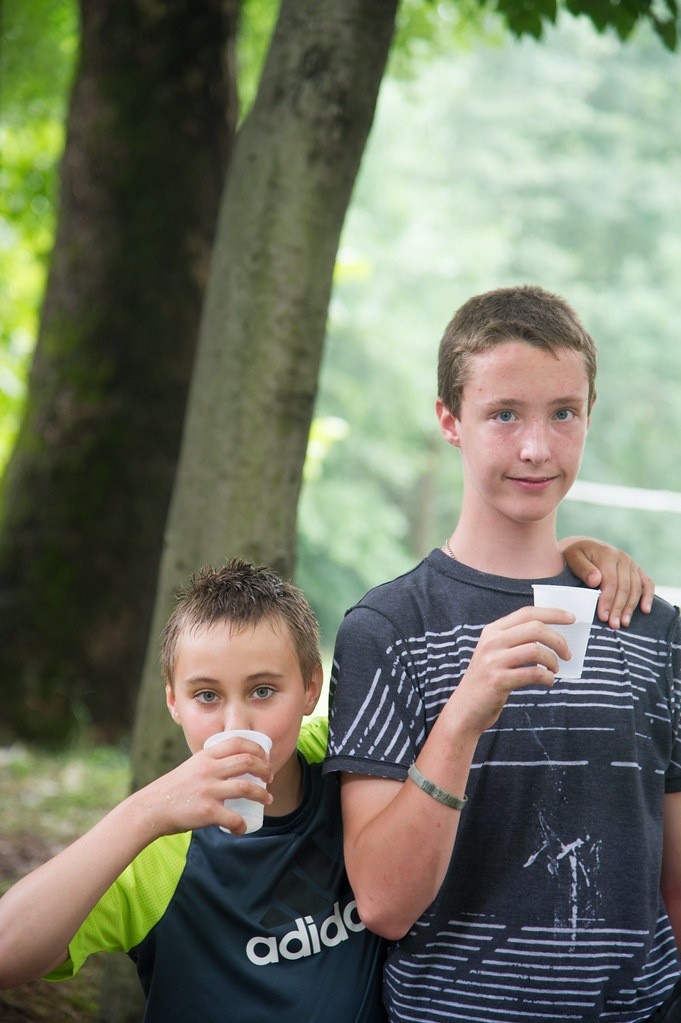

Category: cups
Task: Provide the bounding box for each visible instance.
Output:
[531,583,601,681]
[203,731,273,834]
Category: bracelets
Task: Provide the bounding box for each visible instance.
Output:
[407,764,468,810]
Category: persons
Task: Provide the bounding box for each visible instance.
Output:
[320,286,681,1023]
[0,533,658,1022]
